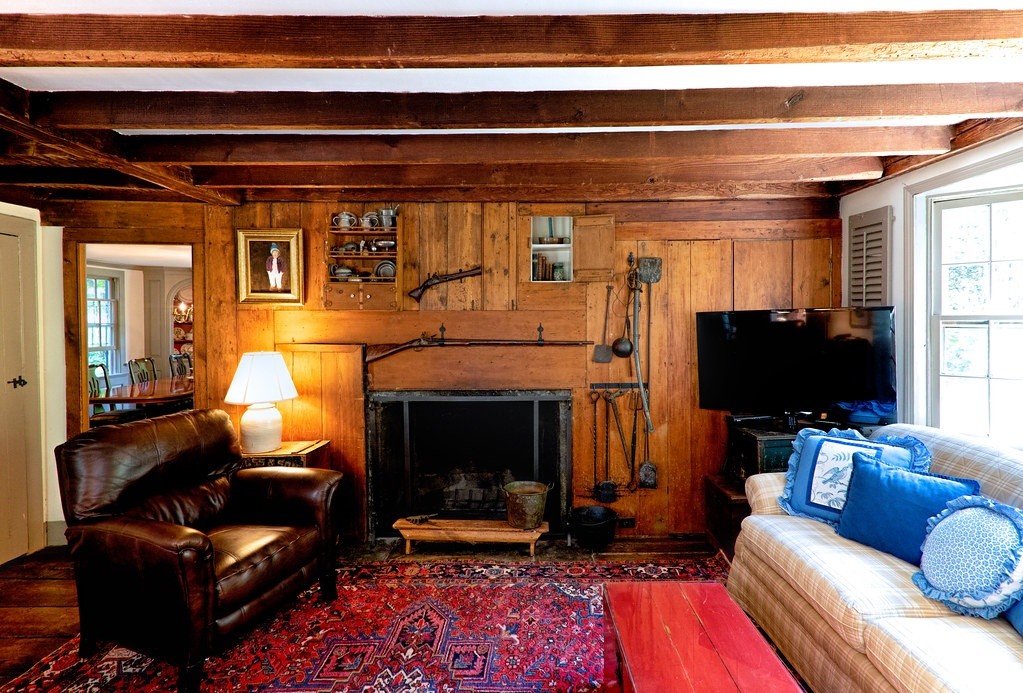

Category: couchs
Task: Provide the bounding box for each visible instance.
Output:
[727,423,1023,693]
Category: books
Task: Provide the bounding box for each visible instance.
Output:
[532,252,554,281]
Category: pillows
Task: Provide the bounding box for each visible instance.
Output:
[910,494,1023,620]
[776,427,932,528]
[830,451,980,569]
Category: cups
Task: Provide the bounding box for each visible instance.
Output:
[533,237,570,244]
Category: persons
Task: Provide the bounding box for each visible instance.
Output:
[265,243,285,293]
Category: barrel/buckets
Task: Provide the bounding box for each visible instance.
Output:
[574,506,617,549]
[498,480,554,529]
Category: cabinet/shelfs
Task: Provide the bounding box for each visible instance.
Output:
[321,216,405,310]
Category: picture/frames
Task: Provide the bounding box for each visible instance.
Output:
[236,227,305,306]
[850,310,872,329]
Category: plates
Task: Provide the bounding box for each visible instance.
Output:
[374,260,396,282]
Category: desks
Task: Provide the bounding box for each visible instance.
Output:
[599,579,807,693]
[703,473,749,561]
[88,372,195,424]
[241,439,331,469]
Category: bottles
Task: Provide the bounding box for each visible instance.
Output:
[533,253,564,281]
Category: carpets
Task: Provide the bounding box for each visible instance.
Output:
[1,547,734,693]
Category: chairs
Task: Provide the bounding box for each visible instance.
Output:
[88,363,116,416]
[168,354,193,408]
[128,355,172,418]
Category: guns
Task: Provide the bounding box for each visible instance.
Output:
[408,266,482,303]
[364,334,594,363]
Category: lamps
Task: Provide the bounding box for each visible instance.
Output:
[223,351,299,453]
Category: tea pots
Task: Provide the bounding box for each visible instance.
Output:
[331,203,399,282]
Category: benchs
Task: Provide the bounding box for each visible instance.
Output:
[51,405,345,693]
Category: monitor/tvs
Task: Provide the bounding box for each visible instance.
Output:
[695,305,898,434]
